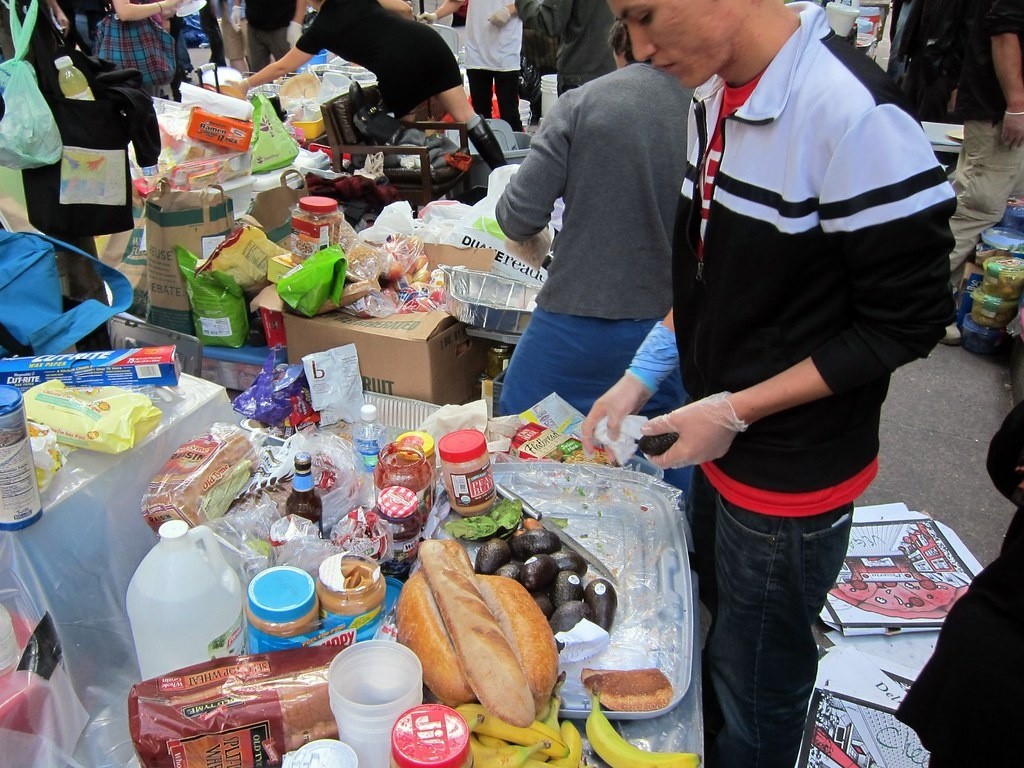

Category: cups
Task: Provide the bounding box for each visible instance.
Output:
[324,639,424,768]
[826,1,859,37]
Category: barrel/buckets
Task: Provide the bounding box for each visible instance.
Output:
[859,6,880,38]
[540,73,558,117]
[519,100,532,133]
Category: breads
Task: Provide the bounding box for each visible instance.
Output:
[396,539,559,728]
[581,667,671,711]
[170,666,339,752]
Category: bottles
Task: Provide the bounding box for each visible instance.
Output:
[387,702,474,768]
[0,385,43,530]
[370,428,496,574]
[286,454,324,541]
[316,553,386,626]
[126,521,247,680]
[352,405,386,481]
[53,56,95,102]
[247,565,319,638]
[290,196,344,264]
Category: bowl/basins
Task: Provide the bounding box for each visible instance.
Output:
[954,200,1024,354]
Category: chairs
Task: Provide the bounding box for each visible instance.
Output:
[320,85,473,218]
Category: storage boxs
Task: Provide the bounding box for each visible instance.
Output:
[281,243,497,407]
[200,346,288,391]
[0,345,181,394]
[170,149,330,221]
[955,263,983,329]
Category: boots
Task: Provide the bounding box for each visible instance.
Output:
[467,114,507,170]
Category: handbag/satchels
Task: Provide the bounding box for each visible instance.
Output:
[349,81,399,144]
[21,98,135,236]
[96,13,176,86]
[144,177,236,310]
[0,229,134,355]
[251,170,310,244]
[0,1,63,169]
[249,92,299,174]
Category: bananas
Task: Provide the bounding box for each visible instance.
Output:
[449,671,701,768]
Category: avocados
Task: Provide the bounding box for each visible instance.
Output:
[475,529,618,645]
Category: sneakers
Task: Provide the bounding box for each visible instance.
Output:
[942,324,962,346]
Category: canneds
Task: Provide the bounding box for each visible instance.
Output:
[373,442,434,525]
[371,486,423,581]
[142,434,257,530]
[0,385,43,532]
[472,373,490,402]
[389,704,474,768]
[395,431,436,498]
[486,344,513,378]
[315,552,386,627]
[438,429,497,517]
[246,566,320,637]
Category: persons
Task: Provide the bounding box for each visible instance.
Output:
[938,0,1024,346]
[420,0,617,133]
[50,0,248,101]
[225,0,507,169]
[583,1,958,768]
[496,21,695,501]
[894,398,1024,768]
[229,0,306,73]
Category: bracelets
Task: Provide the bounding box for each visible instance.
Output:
[158,3,162,12]
[1005,111,1024,115]
[629,321,681,391]
